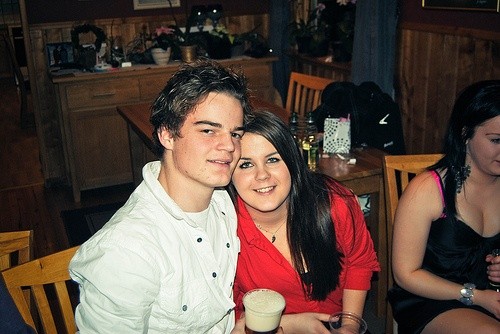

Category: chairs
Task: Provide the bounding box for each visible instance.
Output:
[286,72,336,117]
[0,222,33,311]
[0,244,79,334]
[382,154,447,334]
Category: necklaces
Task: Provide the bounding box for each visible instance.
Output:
[257,222,285,243]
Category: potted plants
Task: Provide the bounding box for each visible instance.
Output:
[205,25,239,60]
[168,15,204,64]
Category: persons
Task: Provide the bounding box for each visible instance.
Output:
[227,111,380,334]
[70,59,285,334]
[391,79,500,334]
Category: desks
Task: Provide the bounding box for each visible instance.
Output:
[319,149,386,319]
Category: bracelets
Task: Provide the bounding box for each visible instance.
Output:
[460,286,473,305]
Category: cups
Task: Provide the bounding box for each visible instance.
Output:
[241,289,286,334]
[327,312,367,334]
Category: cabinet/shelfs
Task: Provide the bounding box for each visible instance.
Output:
[283,49,352,83]
[30,14,277,203]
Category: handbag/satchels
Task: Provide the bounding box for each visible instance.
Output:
[311,81,406,155]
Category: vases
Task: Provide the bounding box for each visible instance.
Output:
[151,47,171,65]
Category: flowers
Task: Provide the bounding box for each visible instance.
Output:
[286,0,358,63]
[148,26,174,50]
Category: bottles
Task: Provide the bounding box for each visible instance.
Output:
[289,112,320,172]
[488,249,500,292]
